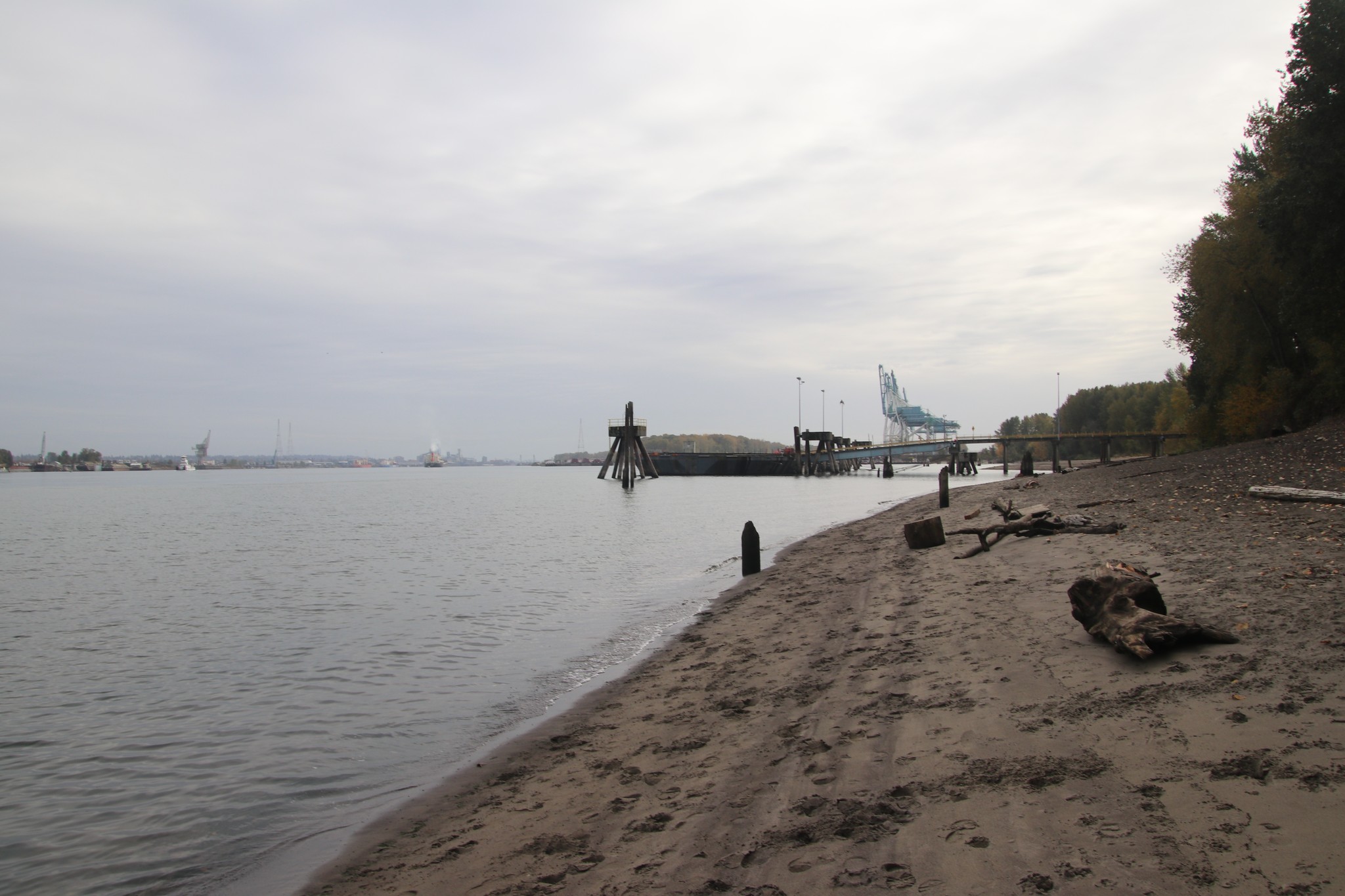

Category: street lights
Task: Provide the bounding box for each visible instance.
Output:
[796,376,845,438]
[1057,372,1060,433]
